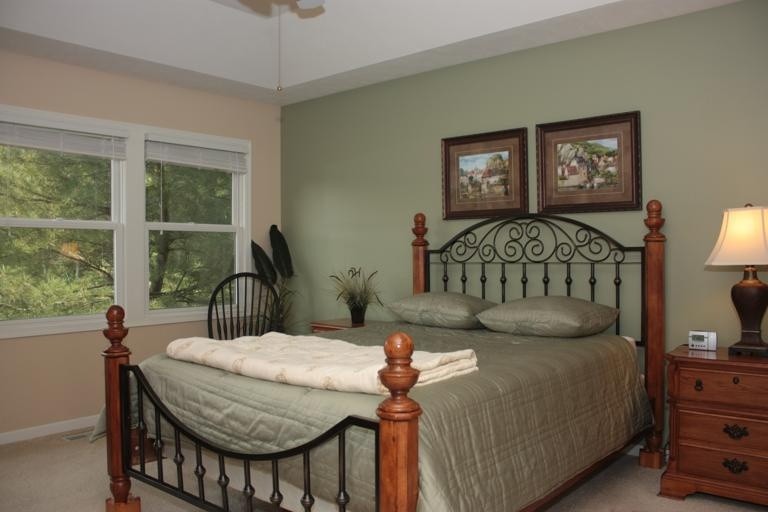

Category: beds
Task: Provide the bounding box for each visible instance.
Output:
[98,197,671,512]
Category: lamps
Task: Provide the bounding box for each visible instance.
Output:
[703,203,768,358]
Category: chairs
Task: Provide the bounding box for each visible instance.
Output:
[205,272,282,340]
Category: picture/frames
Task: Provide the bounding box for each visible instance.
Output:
[439,109,643,222]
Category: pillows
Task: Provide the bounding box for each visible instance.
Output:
[385,290,621,340]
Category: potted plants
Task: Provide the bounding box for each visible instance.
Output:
[326,265,386,325]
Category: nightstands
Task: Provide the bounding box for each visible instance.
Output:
[657,343,768,509]
